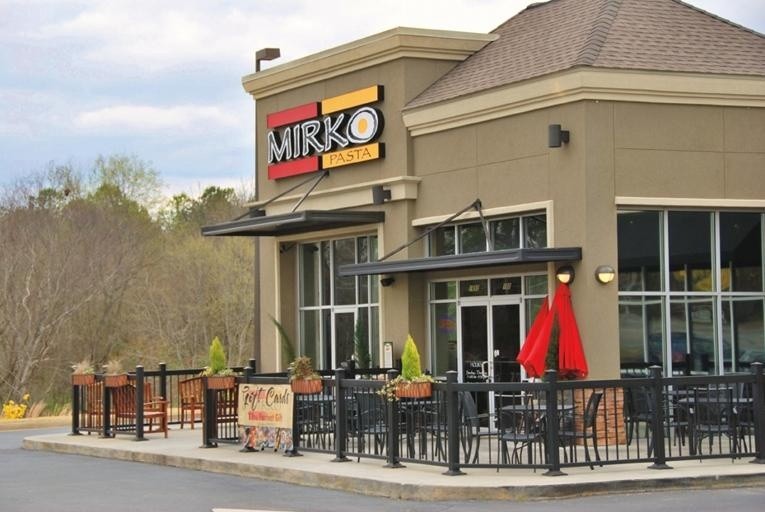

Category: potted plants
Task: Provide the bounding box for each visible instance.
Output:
[287,356,323,394]
[376,334,435,401]
[198,336,236,390]
[69,357,95,386]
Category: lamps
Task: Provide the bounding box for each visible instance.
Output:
[249,208,265,221]
[555,265,575,285]
[547,124,569,149]
[595,265,616,284]
[372,184,392,206]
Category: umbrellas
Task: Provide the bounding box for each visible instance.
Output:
[516,279,586,377]
